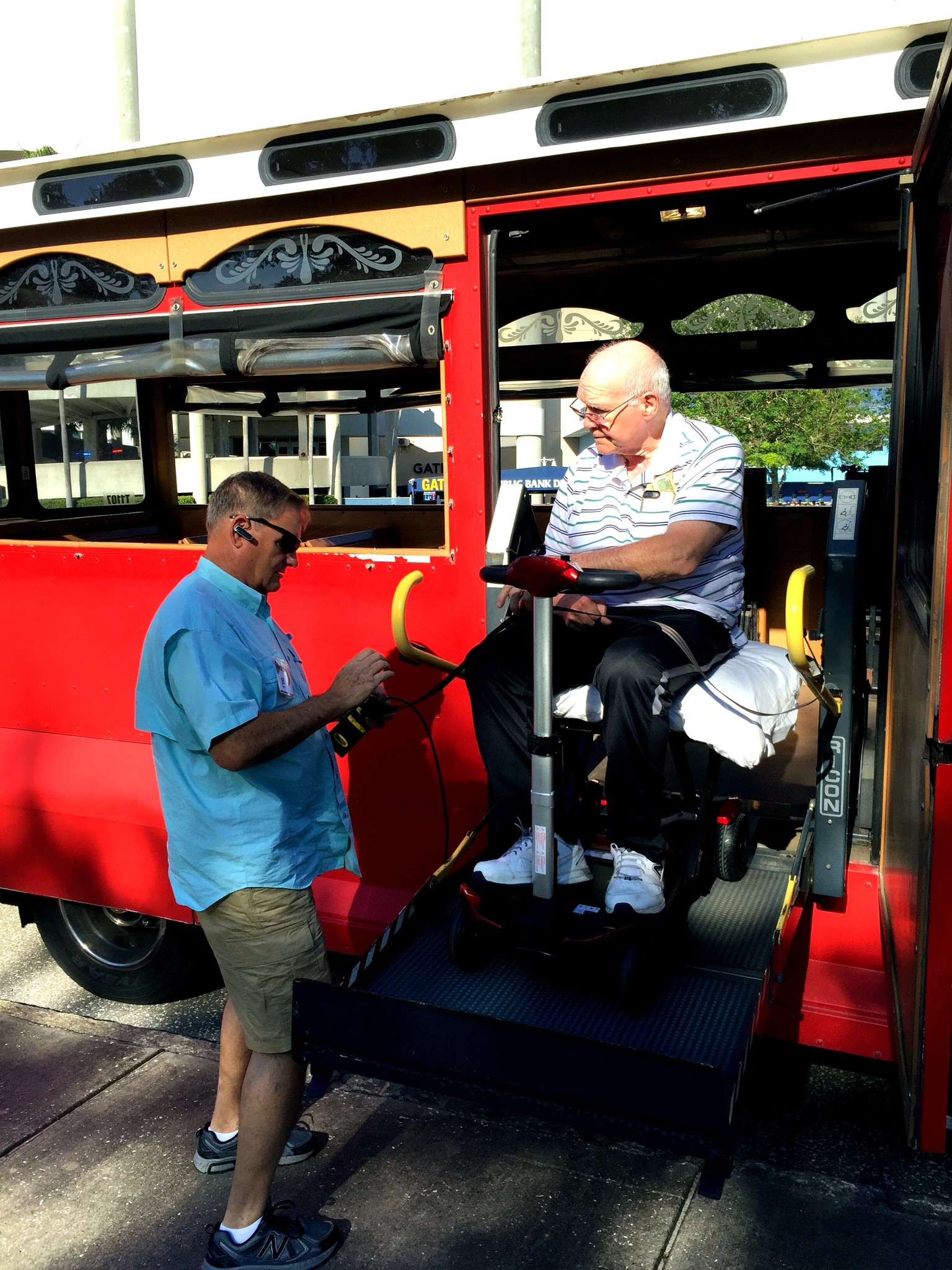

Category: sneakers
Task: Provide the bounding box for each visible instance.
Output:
[200,1200,339,1270]
[194,1120,318,1175]
[605,841,667,915]
[473,823,593,887]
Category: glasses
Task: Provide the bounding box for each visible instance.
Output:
[567,397,638,425]
[230,511,302,556]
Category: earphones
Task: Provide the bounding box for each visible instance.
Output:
[234,525,253,541]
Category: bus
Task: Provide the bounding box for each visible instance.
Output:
[0,20,952,1166]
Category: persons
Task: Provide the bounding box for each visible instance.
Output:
[134,472,394,1266]
[463,339,745,917]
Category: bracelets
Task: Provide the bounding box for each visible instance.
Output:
[561,555,571,562]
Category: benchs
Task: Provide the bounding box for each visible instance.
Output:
[302,529,401,548]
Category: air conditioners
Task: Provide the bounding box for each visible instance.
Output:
[397,438,409,446]
